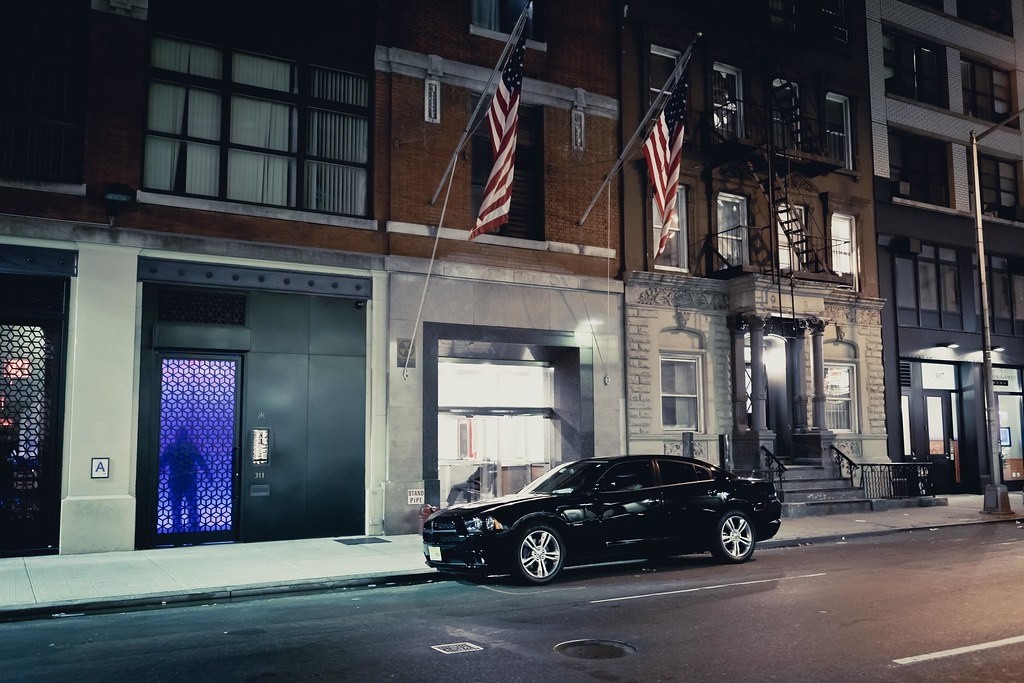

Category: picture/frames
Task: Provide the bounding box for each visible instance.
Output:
[1000,427,1012,447]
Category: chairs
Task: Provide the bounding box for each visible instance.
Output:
[456,466,497,503]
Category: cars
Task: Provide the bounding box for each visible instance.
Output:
[422,454,782,586]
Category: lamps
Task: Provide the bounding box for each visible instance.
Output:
[939,342,959,350]
[763,340,772,364]
[102,182,137,228]
[991,345,1005,353]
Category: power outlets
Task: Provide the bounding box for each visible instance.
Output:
[1012,473,1016,477]
[1016,473,1021,478]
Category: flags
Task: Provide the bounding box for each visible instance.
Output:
[465,2,533,242]
[642,45,693,263]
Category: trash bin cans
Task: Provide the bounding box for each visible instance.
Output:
[418,504,440,535]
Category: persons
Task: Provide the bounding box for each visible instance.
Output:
[160,426,214,547]
[445,458,497,506]
